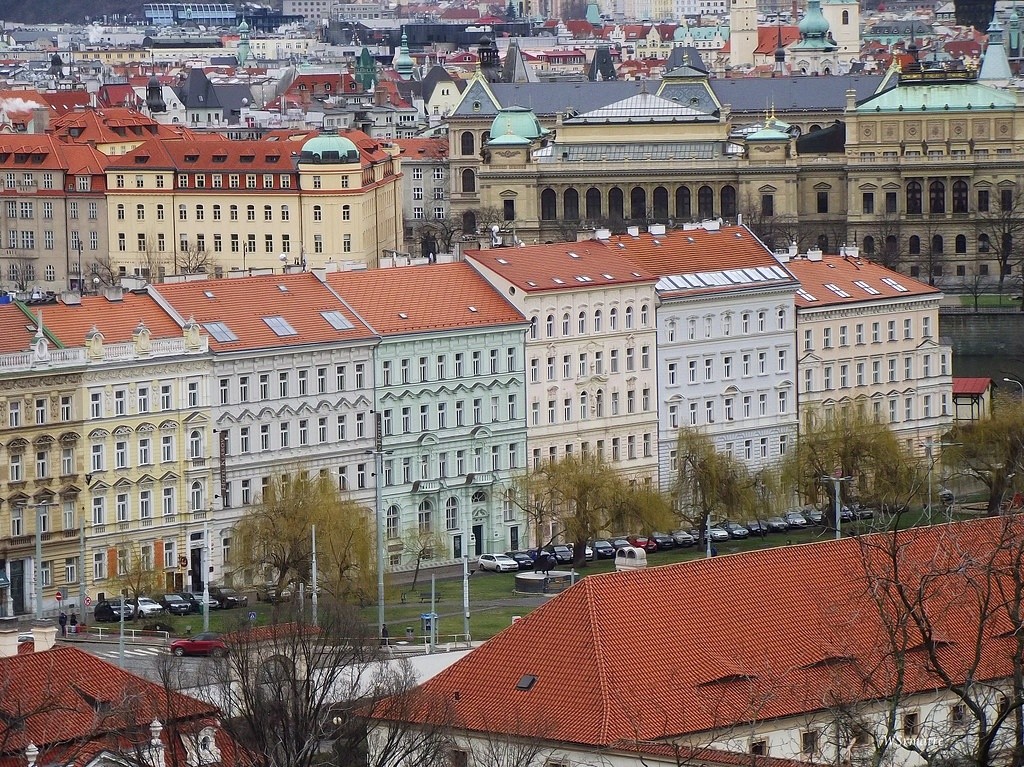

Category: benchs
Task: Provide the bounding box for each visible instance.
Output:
[419,592,443,603]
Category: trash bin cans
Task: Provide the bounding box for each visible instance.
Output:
[406,627,414,642]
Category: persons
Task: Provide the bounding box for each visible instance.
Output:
[70,612,78,632]
[379,624,392,649]
[59,613,67,636]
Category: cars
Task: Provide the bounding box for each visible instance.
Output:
[588,484,954,561]
[94,581,322,623]
[170,632,230,658]
[477,543,594,573]
